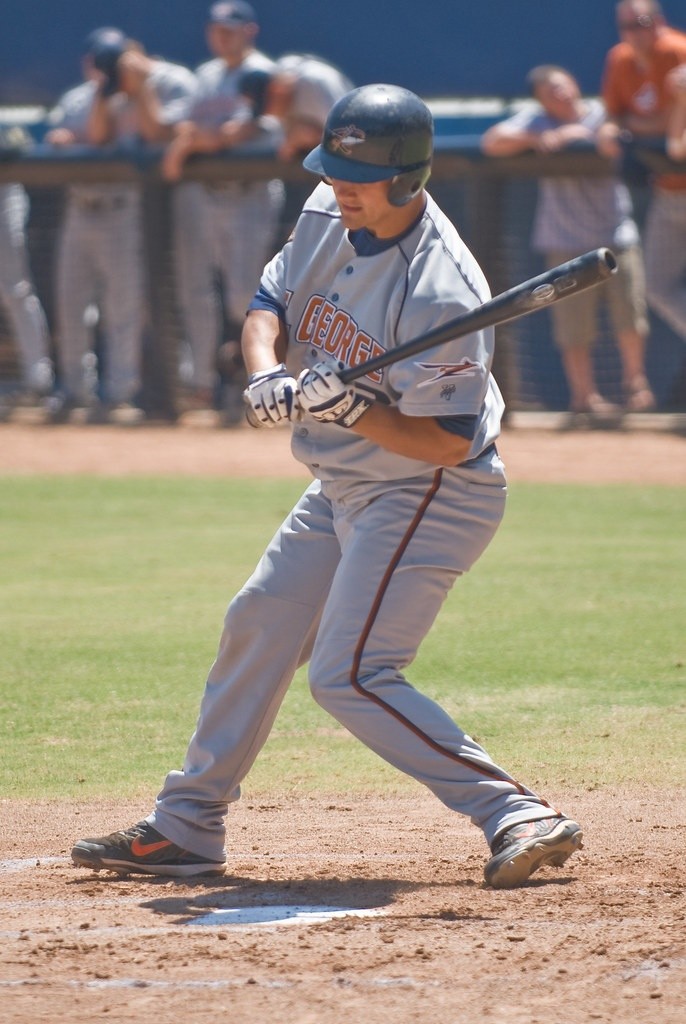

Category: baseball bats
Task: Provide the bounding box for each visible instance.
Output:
[243,245,620,431]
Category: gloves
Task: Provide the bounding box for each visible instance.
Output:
[242,363,299,427]
[296,360,376,429]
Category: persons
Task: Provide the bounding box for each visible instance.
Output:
[67,85,585,889]
[0,0,363,427]
[602,0,686,334]
[479,64,651,429]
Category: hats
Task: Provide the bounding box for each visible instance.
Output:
[202,1,257,26]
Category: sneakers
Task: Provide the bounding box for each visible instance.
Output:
[483,815,583,889]
[71,822,227,877]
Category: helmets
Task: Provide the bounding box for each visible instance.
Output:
[79,26,124,76]
[302,84,435,206]
